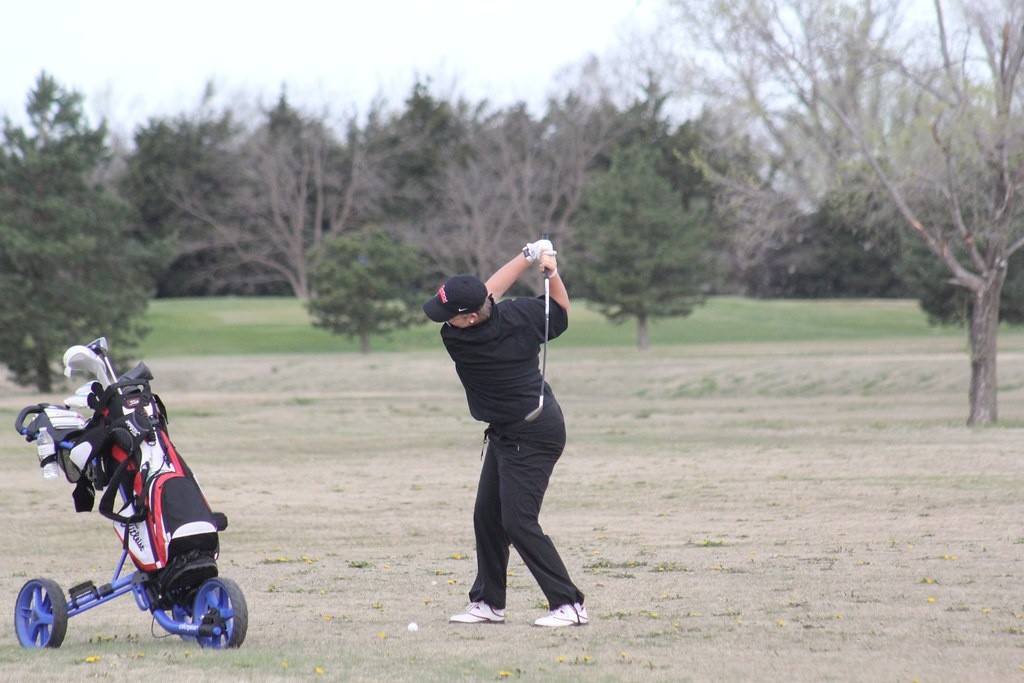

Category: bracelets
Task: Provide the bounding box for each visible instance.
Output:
[543,268,557,278]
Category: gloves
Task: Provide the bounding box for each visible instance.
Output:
[527,239,553,260]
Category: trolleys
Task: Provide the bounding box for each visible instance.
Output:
[11,335,248,653]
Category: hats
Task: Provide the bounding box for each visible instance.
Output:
[423,274,488,322]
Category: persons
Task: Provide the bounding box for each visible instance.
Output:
[424,238,589,628]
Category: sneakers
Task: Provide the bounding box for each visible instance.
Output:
[449,600,505,624]
[535,606,589,627]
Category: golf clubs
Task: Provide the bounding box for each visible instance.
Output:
[62,335,124,412]
[523,231,551,424]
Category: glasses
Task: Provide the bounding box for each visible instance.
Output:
[445,316,464,327]
